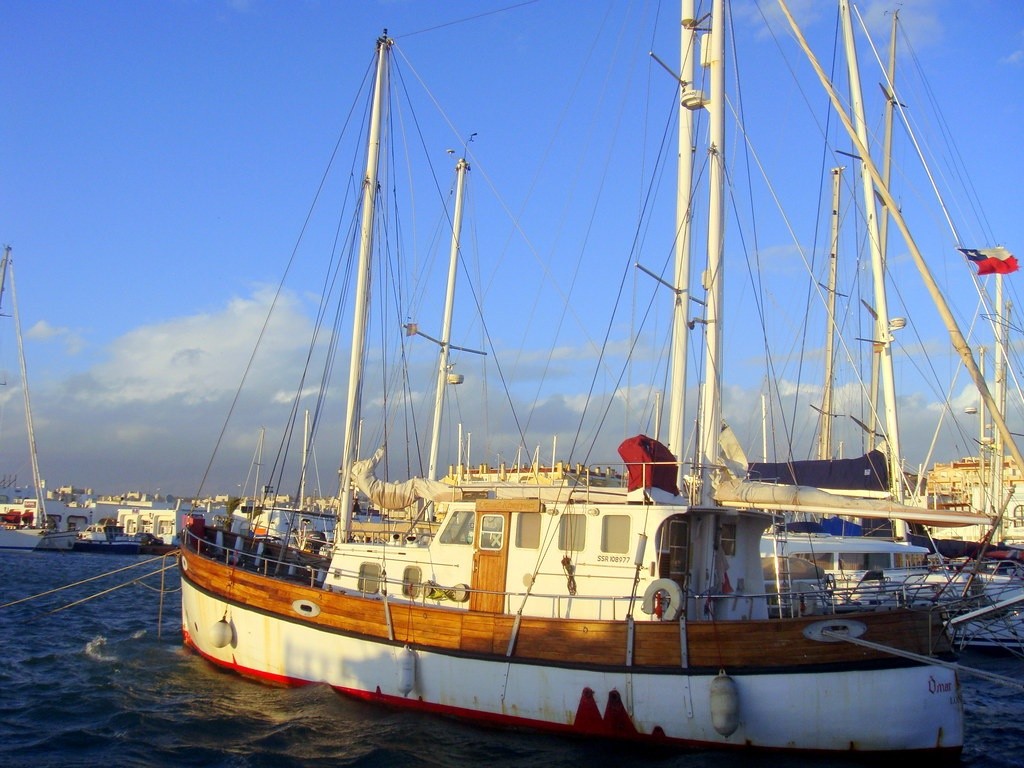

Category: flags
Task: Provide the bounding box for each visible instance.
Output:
[958,246,1020,275]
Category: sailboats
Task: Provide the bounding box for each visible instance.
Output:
[0,243,80,552]
[172,0,1024,768]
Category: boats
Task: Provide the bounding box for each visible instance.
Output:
[72,519,145,554]
[181,511,331,575]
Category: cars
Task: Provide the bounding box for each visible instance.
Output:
[133,532,164,545]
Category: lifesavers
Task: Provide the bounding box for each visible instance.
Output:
[641,577,684,621]
[1010,627,1023,641]
